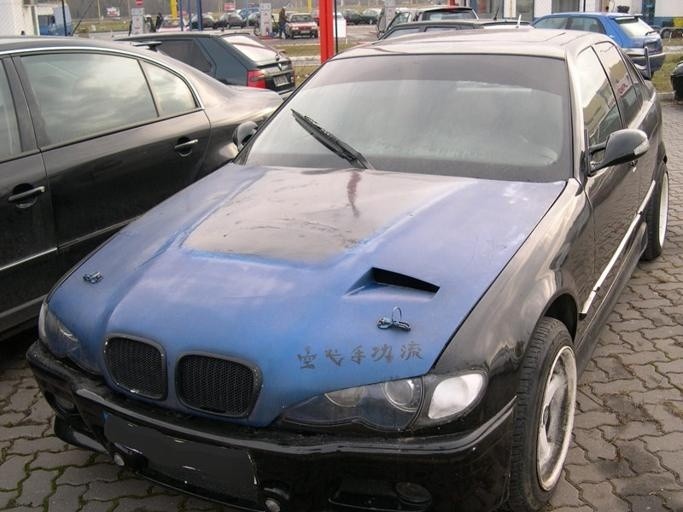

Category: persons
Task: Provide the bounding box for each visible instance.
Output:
[155,12,163,32]
[278,6,290,40]
